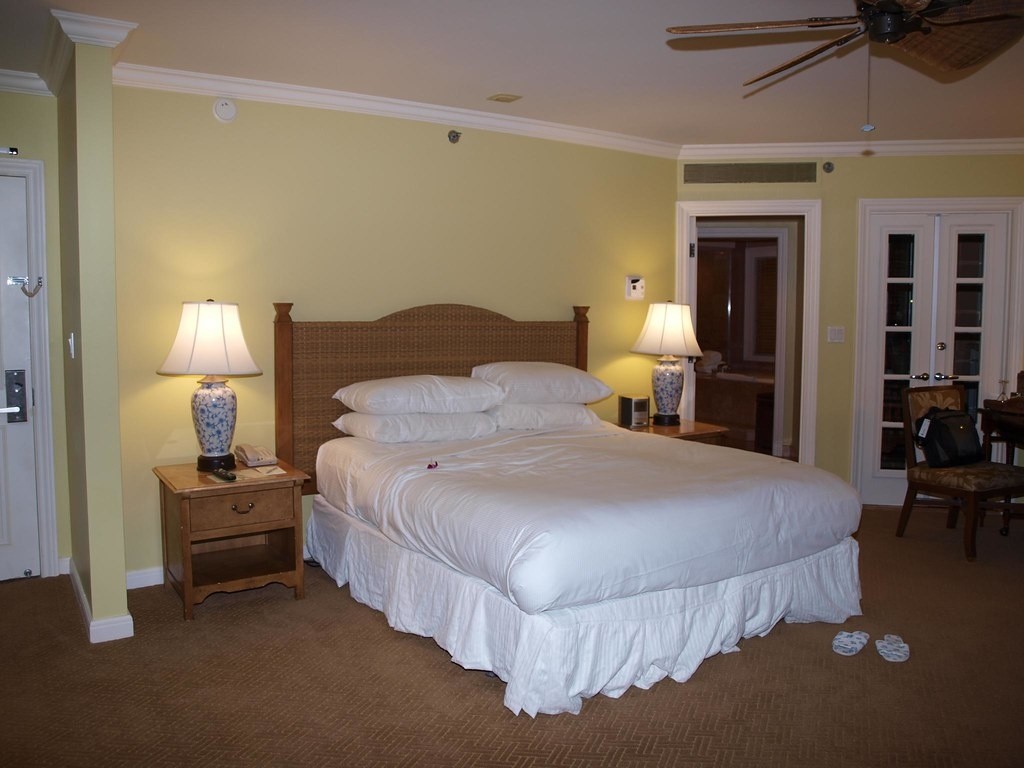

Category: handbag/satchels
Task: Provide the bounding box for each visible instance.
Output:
[913,406,991,468]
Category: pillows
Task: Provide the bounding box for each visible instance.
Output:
[489,405,603,429]
[472,361,614,403]
[331,412,497,444]
[332,374,503,415]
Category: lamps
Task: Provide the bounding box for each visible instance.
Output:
[629,302,704,426]
[156,298,263,471]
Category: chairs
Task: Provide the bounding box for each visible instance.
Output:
[895,386,1024,562]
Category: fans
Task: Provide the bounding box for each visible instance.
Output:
[667,0,1024,132]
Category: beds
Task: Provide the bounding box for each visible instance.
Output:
[272,301,862,718]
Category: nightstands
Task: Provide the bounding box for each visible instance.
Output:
[153,460,311,619]
[617,422,730,446]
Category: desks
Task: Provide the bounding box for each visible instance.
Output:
[975,407,1024,525]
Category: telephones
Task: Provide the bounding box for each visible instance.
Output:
[234,443,278,467]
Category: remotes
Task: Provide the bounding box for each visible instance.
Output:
[213,468,236,481]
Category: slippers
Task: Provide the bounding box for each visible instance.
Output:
[832,630,869,656]
[874,634,909,662]
